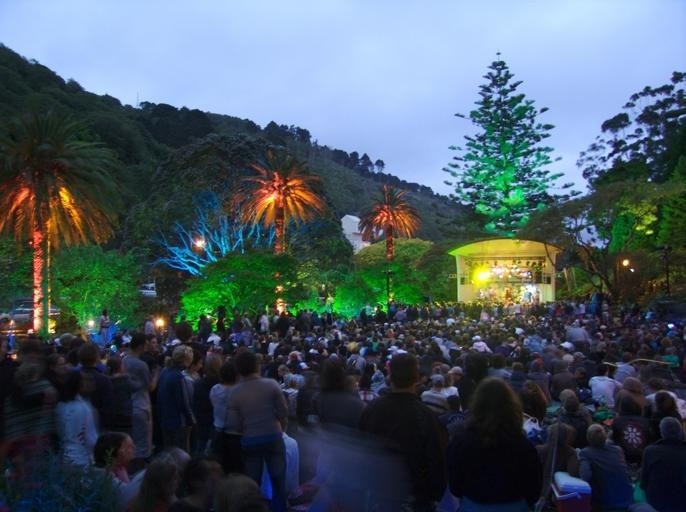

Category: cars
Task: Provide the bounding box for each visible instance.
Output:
[0,299,59,325]
[138,282,155,297]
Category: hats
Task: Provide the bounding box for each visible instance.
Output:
[297,361,309,371]
[559,387,579,411]
[560,341,575,353]
[430,364,463,385]
[472,335,482,341]
[348,341,359,352]
